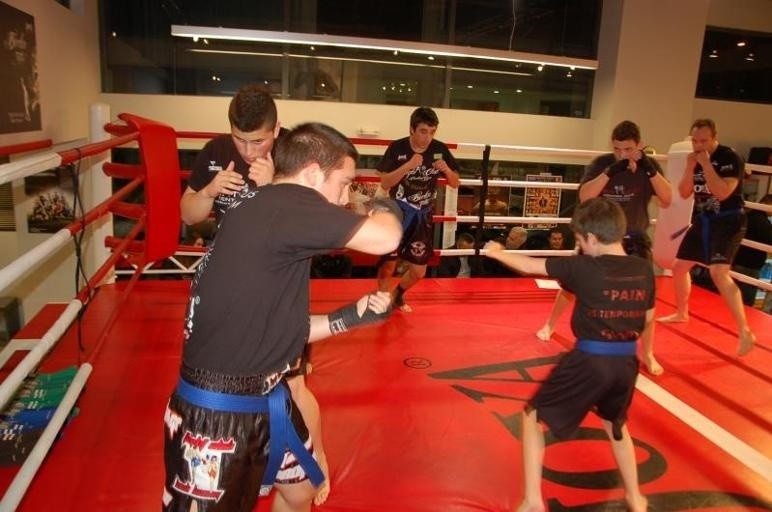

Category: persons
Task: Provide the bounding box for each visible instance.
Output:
[375,107,461,314]
[0,3,31,132]
[655,116,757,360]
[177,227,568,276]
[294,56,338,102]
[178,86,331,508]
[31,190,73,222]
[478,195,658,512]
[706,192,771,309]
[161,120,404,511]
[534,118,675,379]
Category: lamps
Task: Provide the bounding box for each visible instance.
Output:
[170,0,599,71]
